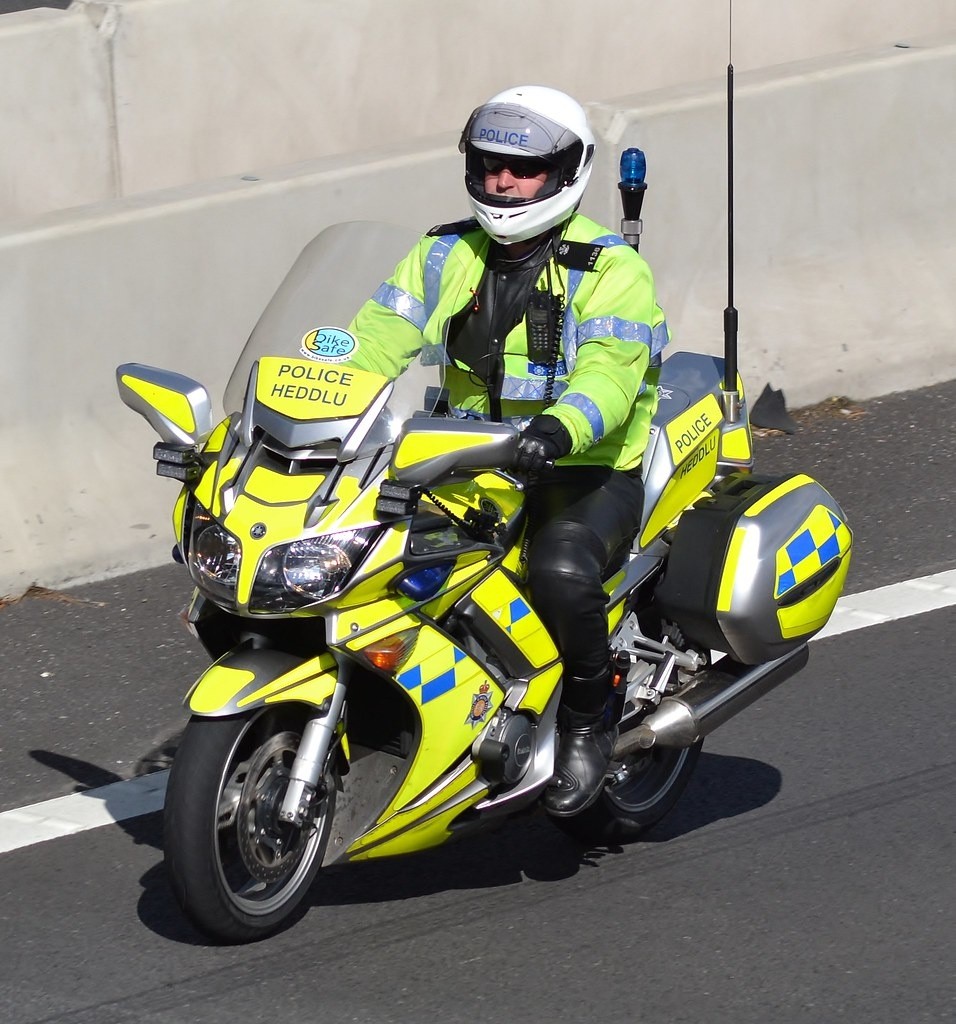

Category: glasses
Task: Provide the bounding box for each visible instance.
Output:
[480,152,557,179]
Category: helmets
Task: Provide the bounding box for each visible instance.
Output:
[465,86,597,245]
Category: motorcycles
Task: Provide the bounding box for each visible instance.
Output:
[113,220,855,944]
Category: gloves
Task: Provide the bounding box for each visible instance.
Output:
[506,415,569,483]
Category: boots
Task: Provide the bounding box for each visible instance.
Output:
[537,664,618,815]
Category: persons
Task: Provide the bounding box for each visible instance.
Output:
[329,86,670,817]
